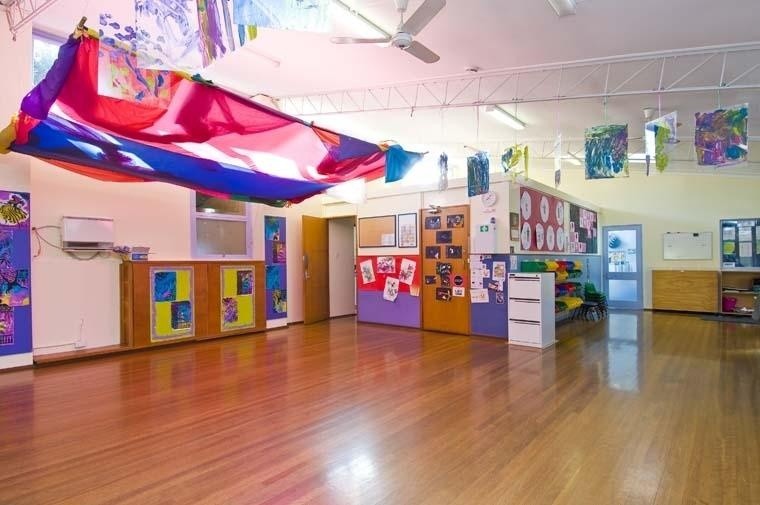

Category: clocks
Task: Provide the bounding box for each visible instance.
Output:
[480,188,496,207]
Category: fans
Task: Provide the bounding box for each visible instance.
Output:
[330,1,447,65]
[627,106,683,159]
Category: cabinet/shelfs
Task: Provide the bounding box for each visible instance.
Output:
[504,269,556,348]
[650,268,760,319]
[122,260,268,351]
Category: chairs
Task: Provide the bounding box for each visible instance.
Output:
[566,279,610,322]
